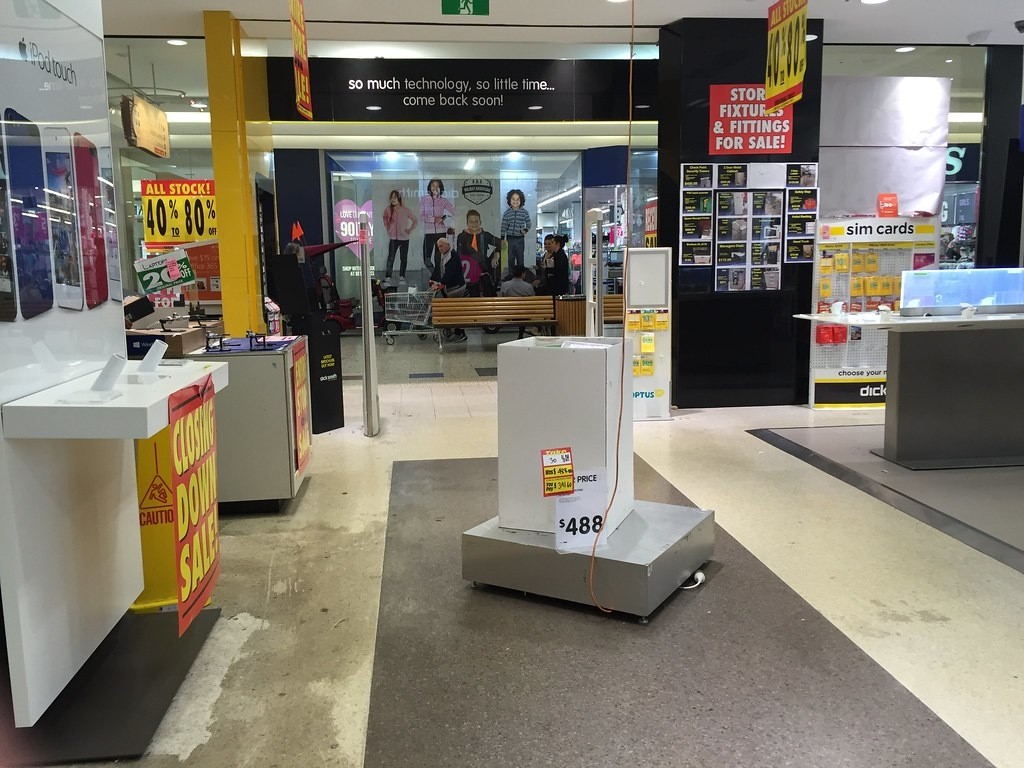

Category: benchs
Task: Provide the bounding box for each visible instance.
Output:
[430,291,622,353]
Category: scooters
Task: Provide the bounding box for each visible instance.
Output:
[317,266,401,336]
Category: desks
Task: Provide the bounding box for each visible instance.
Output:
[793,310,1024,471]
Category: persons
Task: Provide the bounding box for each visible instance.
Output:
[500,187,532,282]
[429,237,468,343]
[525,232,570,336]
[497,265,542,341]
[457,209,501,291]
[382,191,419,288]
[419,177,455,285]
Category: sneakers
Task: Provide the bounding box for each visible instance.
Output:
[384,277,391,287]
[399,276,407,286]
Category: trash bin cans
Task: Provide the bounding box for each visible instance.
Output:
[555,294,585,336]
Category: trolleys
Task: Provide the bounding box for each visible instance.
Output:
[382,284,445,345]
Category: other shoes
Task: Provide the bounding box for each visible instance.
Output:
[459,336,468,342]
[449,335,461,341]
